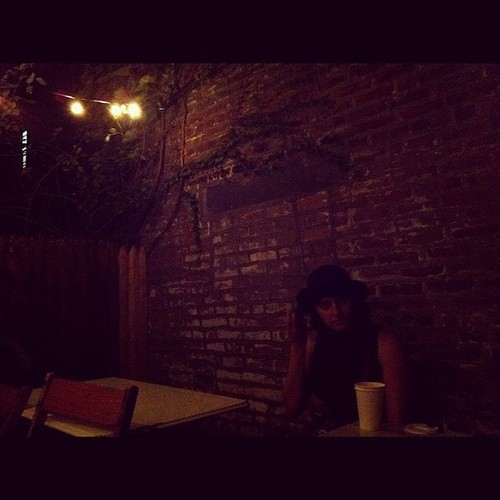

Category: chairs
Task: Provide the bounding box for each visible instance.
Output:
[1,381,31,456]
[0,369,140,500]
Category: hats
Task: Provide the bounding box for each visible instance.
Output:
[296,265,370,306]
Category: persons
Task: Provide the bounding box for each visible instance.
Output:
[282,262,407,429]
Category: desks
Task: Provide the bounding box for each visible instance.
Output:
[316,420,468,500]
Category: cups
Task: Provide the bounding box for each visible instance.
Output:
[355,380,387,434]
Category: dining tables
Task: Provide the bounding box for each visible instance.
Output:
[12,376,249,500]
